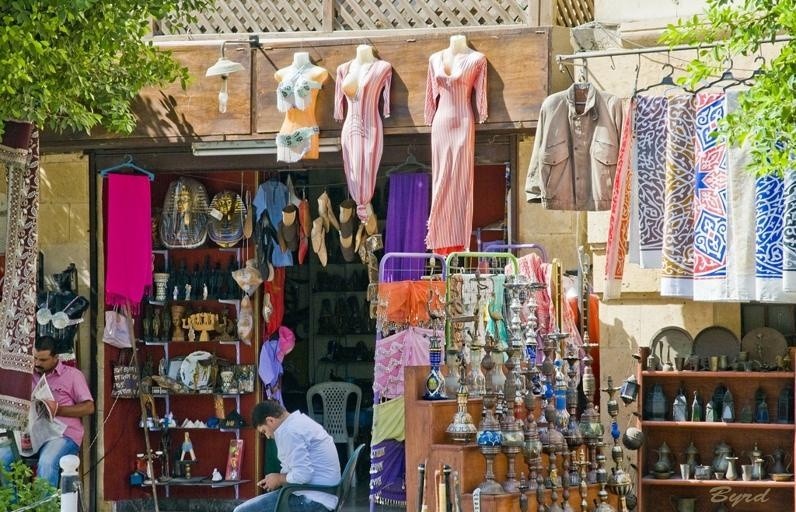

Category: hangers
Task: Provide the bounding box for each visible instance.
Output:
[723,56,772,92]
[385,144,432,178]
[100,153,155,182]
[695,58,754,92]
[268,168,349,200]
[574,65,590,104]
[631,64,695,99]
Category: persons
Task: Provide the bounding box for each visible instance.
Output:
[233,400,342,511]
[333,45,394,226]
[275,51,329,161]
[0,334,95,505]
[423,32,489,257]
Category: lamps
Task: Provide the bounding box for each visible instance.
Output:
[204,36,258,114]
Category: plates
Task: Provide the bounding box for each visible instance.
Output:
[180,350,213,391]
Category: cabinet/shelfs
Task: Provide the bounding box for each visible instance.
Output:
[404,364,629,512]
[638,346,796,512]
[141,247,252,500]
[309,219,387,385]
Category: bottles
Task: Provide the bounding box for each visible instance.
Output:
[443,392,477,445]
[716,501,731,511]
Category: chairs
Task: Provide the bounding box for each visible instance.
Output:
[306,382,363,488]
[274,444,366,512]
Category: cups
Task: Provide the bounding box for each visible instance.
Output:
[647,344,793,371]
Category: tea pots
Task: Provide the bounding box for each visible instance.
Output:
[647,442,794,480]
[666,493,704,512]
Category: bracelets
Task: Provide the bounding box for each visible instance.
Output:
[56,405,64,415]
